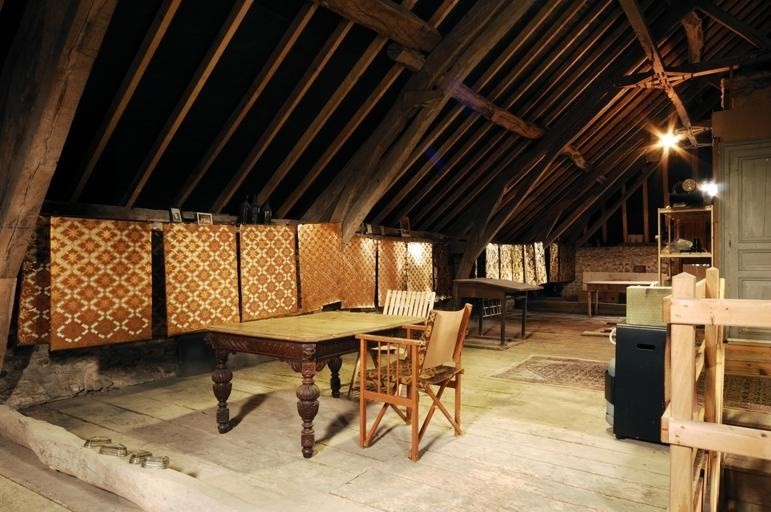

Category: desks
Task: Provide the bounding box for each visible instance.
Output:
[452,277,547,351]
[203,309,432,459]
[584,280,660,319]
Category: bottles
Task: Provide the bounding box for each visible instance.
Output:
[240,193,272,224]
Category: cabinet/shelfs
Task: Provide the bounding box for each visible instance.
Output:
[657,204,718,286]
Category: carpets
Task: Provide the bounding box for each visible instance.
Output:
[484,354,611,393]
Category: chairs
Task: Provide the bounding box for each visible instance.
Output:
[352,302,476,461]
[346,286,440,404]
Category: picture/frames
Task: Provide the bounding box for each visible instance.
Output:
[196,212,212,225]
[169,207,183,223]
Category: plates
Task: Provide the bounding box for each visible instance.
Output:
[83,436,170,469]
